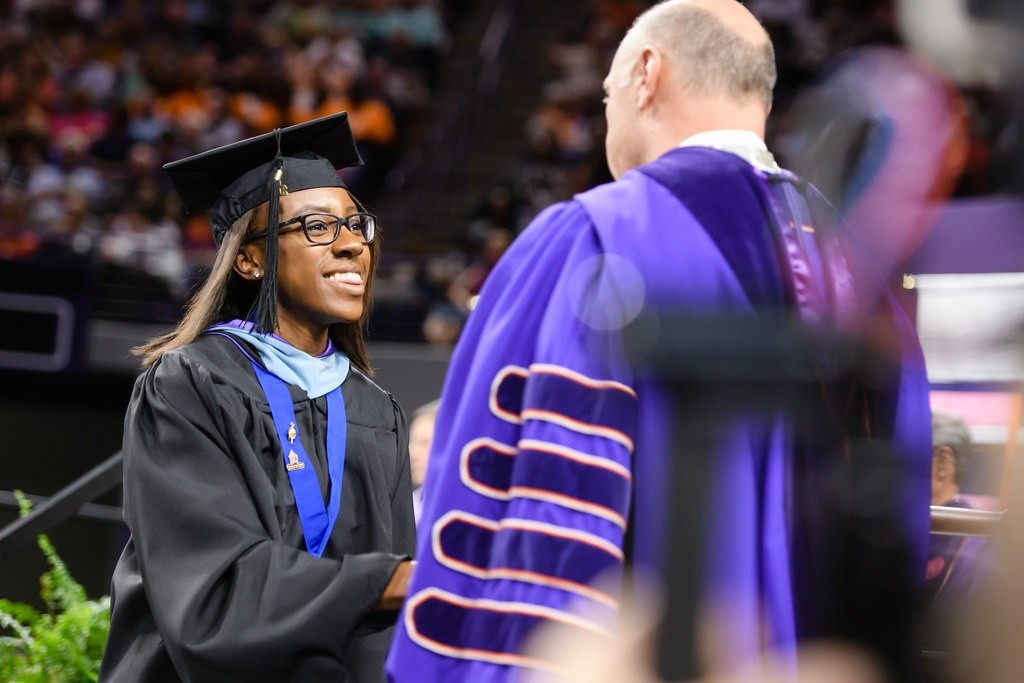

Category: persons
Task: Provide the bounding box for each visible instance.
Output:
[385,0,929,683]
[1,0,626,346]
[102,109,416,683]
[921,404,984,600]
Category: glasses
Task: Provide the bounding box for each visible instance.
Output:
[237,213,377,249]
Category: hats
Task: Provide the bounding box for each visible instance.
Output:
[164,110,363,252]
